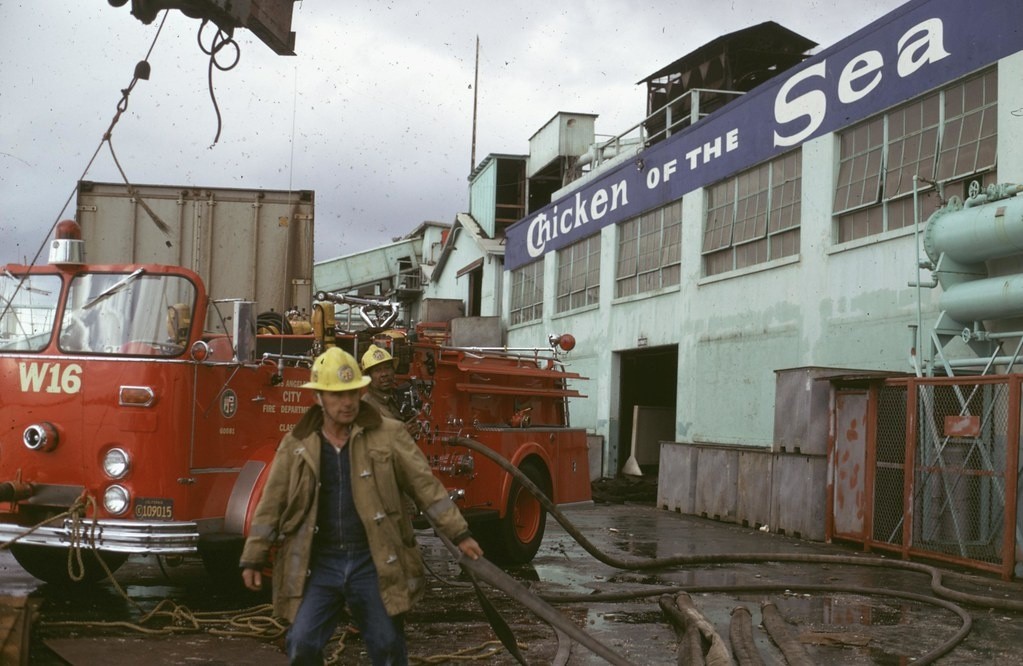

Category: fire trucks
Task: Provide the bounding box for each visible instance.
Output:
[0,220,591,608]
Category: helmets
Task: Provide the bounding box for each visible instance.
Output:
[361,345,397,373]
[298,347,372,391]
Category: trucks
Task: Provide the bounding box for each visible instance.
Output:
[75,180,316,340]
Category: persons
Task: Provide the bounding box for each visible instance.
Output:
[240,348,483,666]
[346,344,421,636]
